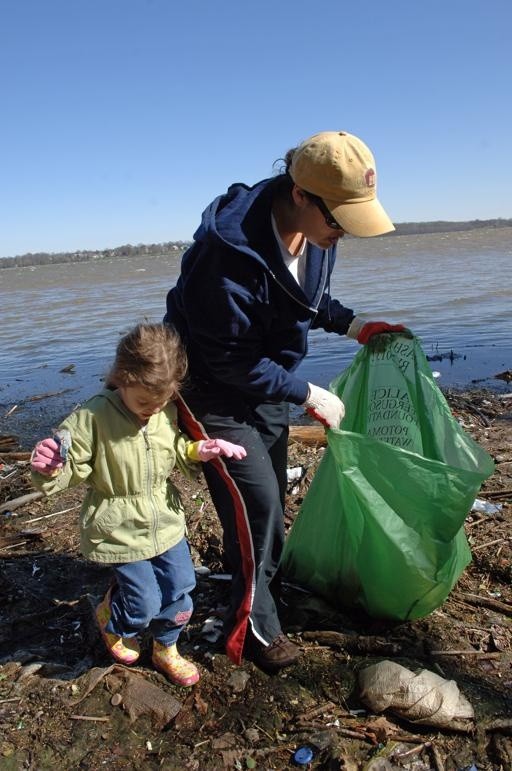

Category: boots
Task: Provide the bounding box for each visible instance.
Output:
[151,639,200,689]
[94,584,141,665]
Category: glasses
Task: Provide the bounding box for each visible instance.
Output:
[313,196,343,230]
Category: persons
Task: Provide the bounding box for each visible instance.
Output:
[161,131,413,671]
[29,322,247,687]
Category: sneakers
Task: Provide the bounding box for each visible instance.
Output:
[256,630,304,669]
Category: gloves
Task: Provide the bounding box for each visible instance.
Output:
[30,438,65,474]
[196,439,247,464]
[301,381,347,431]
[346,316,415,352]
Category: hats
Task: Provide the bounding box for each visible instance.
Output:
[287,128,397,239]
